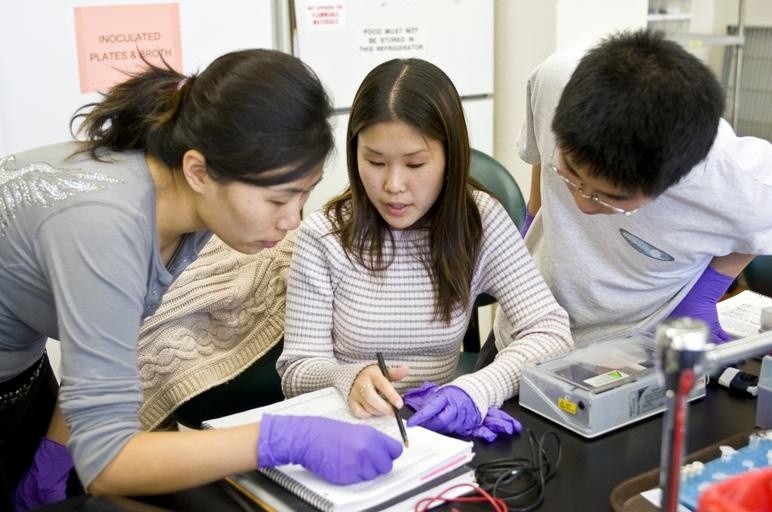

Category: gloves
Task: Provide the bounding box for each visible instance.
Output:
[522,208,534,240]
[401,381,522,442]
[12,437,74,512]
[669,266,734,345]
[257,412,403,486]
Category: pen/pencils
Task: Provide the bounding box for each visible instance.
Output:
[376,352,408,447]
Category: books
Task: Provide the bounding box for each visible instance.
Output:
[201,386,475,512]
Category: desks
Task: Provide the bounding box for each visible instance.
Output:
[23,353,772,512]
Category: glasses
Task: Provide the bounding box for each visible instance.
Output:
[544,143,640,218]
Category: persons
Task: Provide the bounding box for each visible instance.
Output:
[276,58,574,437]
[0,49,403,512]
[473,25,772,373]
